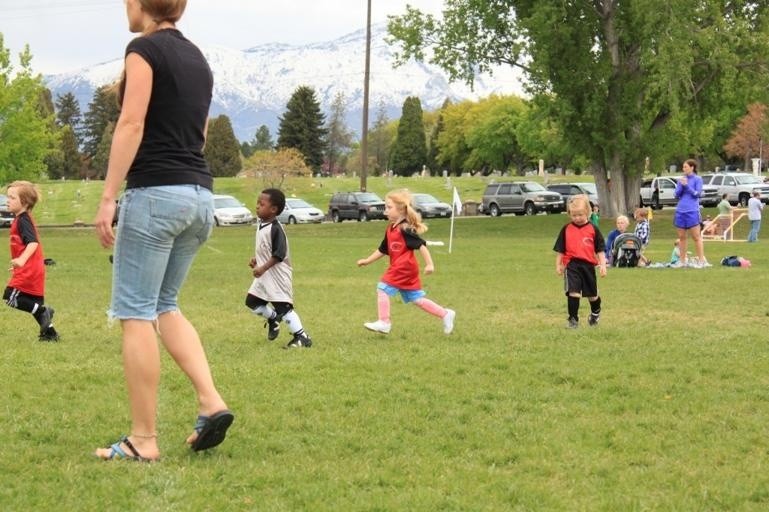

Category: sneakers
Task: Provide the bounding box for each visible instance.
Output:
[39,307,58,340]
[268,310,280,340]
[569,318,579,328]
[588,316,599,326]
[443,310,455,334]
[364,320,391,333]
[287,335,312,347]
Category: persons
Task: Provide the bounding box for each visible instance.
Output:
[552,194,605,326]
[588,187,765,267]
[673,159,709,268]
[245,188,313,346]
[3,180,59,342]
[354,188,456,335]
[94,1,234,460]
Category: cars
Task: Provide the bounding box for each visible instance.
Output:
[0,194,16,228]
[410,192,453,219]
[213,194,253,227]
[276,198,326,225]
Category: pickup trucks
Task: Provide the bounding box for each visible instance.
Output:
[640,176,720,210]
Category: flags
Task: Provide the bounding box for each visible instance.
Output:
[455,189,462,216]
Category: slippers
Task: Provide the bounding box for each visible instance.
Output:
[95,436,162,461]
[191,410,234,452]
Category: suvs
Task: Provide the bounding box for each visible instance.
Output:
[481,180,566,218]
[699,172,769,207]
[545,182,600,217]
[329,190,388,223]
